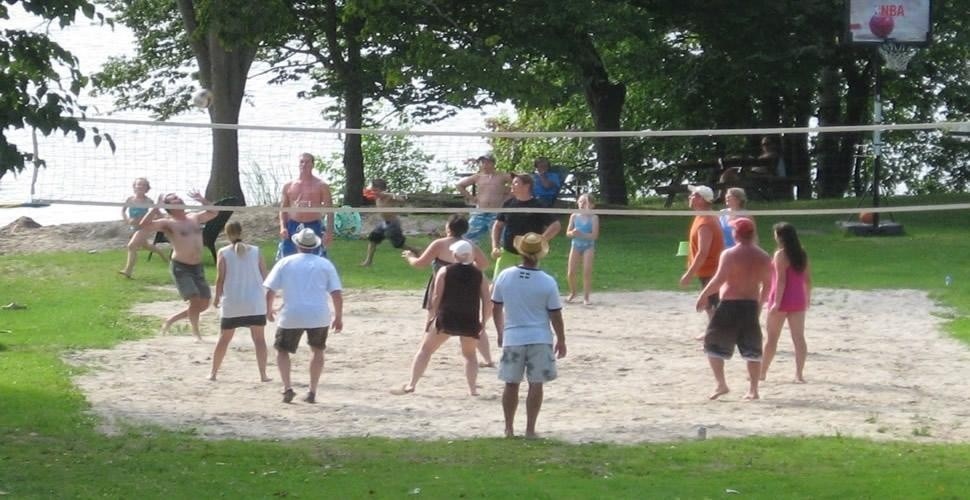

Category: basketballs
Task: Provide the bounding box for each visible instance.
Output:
[860,211,871,221]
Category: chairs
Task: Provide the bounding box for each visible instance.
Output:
[147,196,239,264]
[540,166,568,224]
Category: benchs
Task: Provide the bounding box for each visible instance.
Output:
[656,156,795,208]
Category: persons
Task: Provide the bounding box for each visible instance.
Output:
[492,231,566,438]
[401,241,481,396]
[529,156,561,207]
[400,214,495,367]
[115,176,274,383]
[745,222,812,384]
[488,171,563,293]
[713,136,778,204]
[679,184,726,342]
[696,218,773,401]
[263,227,343,404]
[275,152,334,261]
[455,154,512,247]
[564,193,600,306]
[717,187,748,248]
[358,179,420,267]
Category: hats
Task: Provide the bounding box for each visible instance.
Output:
[688,184,713,203]
[481,154,494,160]
[729,218,753,234]
[514,232,549,262]
[291,228,321,250]
[510,173,531,183]
[450,240,472,256]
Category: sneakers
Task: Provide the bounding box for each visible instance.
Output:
[282,389,294,402]
[303,391,314,403]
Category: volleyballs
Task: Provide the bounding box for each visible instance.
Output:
[196,89,214,109]
